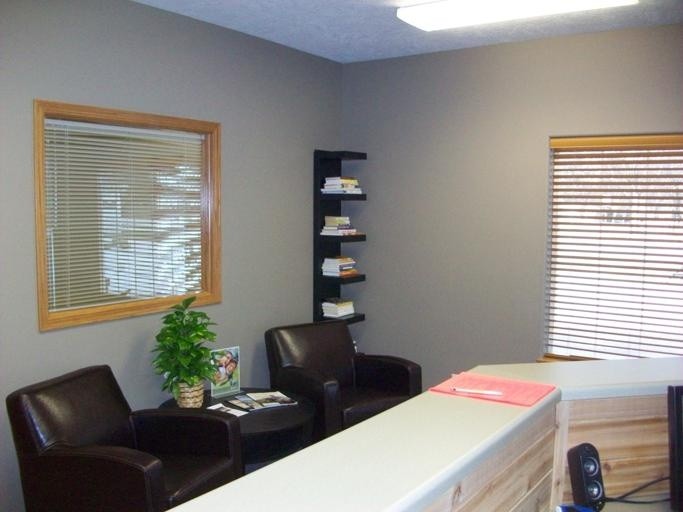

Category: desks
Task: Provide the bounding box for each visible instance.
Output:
[158,386,318,462]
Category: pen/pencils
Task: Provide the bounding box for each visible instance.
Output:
[454,387,502,395]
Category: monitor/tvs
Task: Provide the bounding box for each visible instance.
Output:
[668,384,683,512]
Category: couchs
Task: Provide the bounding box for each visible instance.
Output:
[5,363,245,512]
[264,317,424,437]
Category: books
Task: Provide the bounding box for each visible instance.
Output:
[234,395,267,410]
[320,176,362,195]
[223,398,257,411]
[319,215,357,237]
[320,297,354,319]
[320,255,357,277]
[205,402,249,418]
[245,390,297,408]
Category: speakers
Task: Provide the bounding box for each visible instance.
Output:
[567,442,606,512]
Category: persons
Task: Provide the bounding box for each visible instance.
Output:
[219,358,237,386]
[214,351,233,383]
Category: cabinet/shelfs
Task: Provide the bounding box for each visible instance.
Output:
[312,146,370,329]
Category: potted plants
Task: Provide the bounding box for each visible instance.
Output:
[150,296,227,414]
